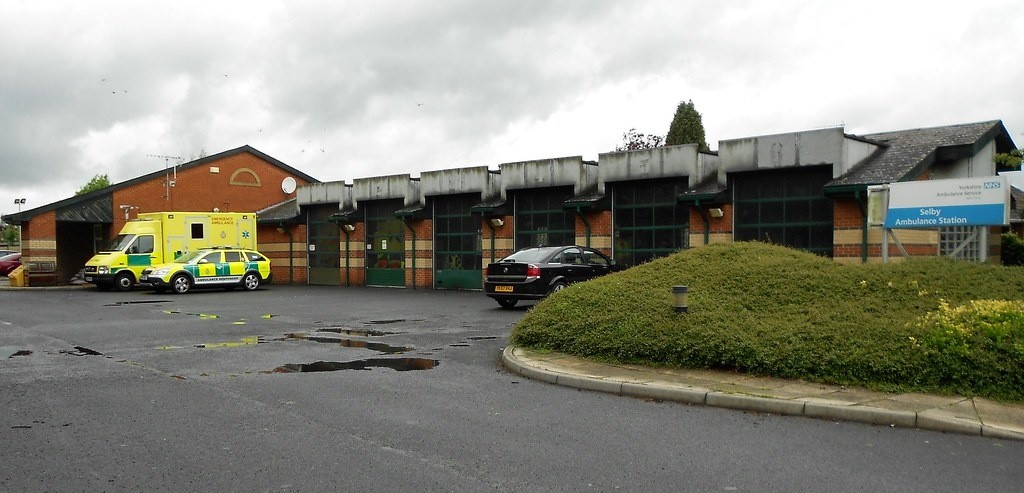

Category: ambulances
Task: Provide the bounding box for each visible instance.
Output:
[138,246,273,291]
[83,210,258,291]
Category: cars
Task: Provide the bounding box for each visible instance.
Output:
[483,245,623,308]
